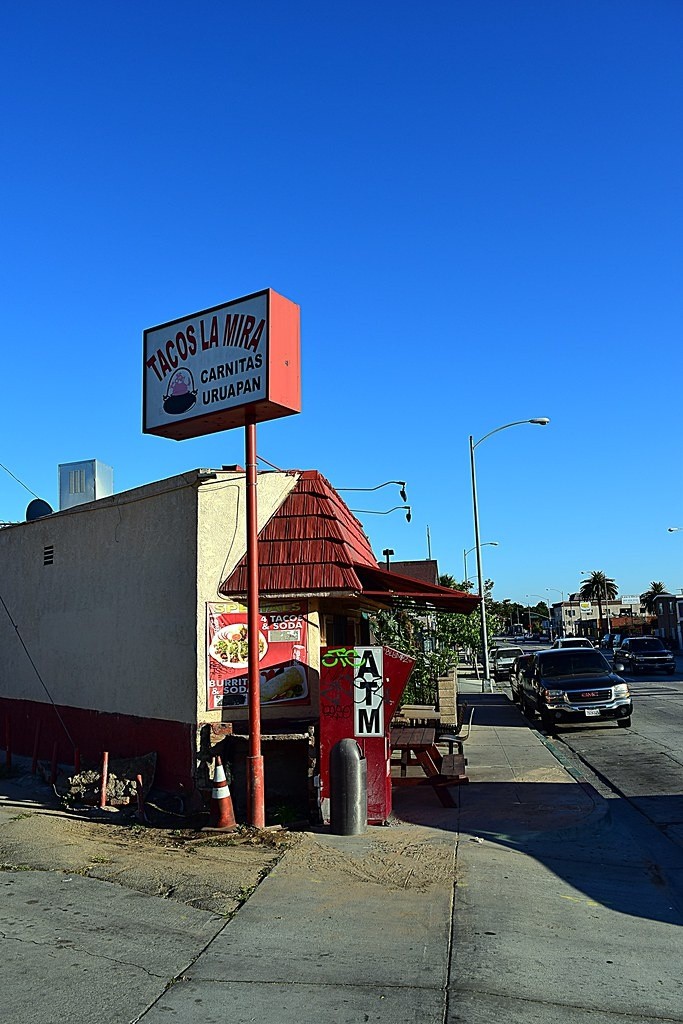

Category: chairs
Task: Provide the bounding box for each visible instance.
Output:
[440,700,475,766]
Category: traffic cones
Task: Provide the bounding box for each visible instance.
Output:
[201,756,239,833]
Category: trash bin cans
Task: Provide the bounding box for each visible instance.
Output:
[328,738,368,837]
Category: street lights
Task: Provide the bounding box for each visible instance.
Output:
[546,588,566,638]
[468,413,555,695]
[525,594,554,642]
[581,569,611,634]
[463,542,500,592]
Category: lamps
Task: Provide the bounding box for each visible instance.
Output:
[349,506,411,522]
[335,481,407,502]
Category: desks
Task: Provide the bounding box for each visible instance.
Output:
[401,705,441,726]
[389,728,456,808]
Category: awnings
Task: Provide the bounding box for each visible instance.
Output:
[352,558,484,615]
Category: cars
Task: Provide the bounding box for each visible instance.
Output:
[549,637,600,650]
[585,632,633,654]
[612,635,677,676]
[509,653,532,703]
[519,646,635,733]
[514,633,526,643]
[489,645,526,676]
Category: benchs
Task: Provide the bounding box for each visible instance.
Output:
[440,753,466,779]
